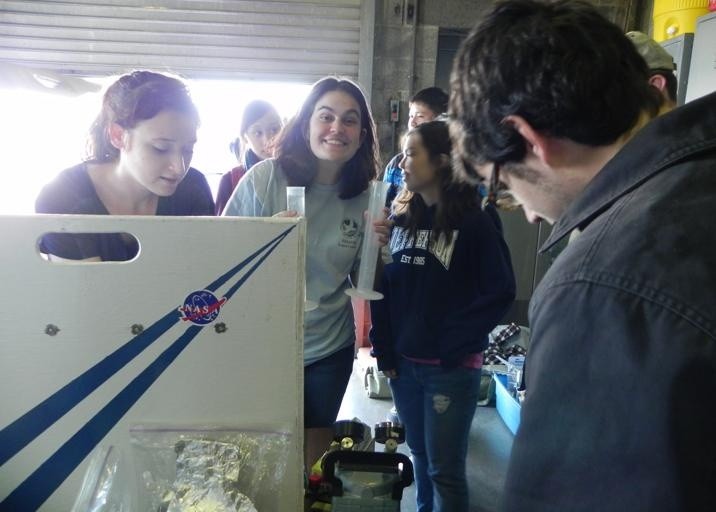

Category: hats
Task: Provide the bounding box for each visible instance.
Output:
[624,30,681,70]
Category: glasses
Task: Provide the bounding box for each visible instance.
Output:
[481,161,520,213]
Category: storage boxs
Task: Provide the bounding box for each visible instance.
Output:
[492,371,523,438]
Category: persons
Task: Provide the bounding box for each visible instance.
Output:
[34,68,217,265]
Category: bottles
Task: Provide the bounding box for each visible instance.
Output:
[284,183,321,315]
[344,178,393,303]
